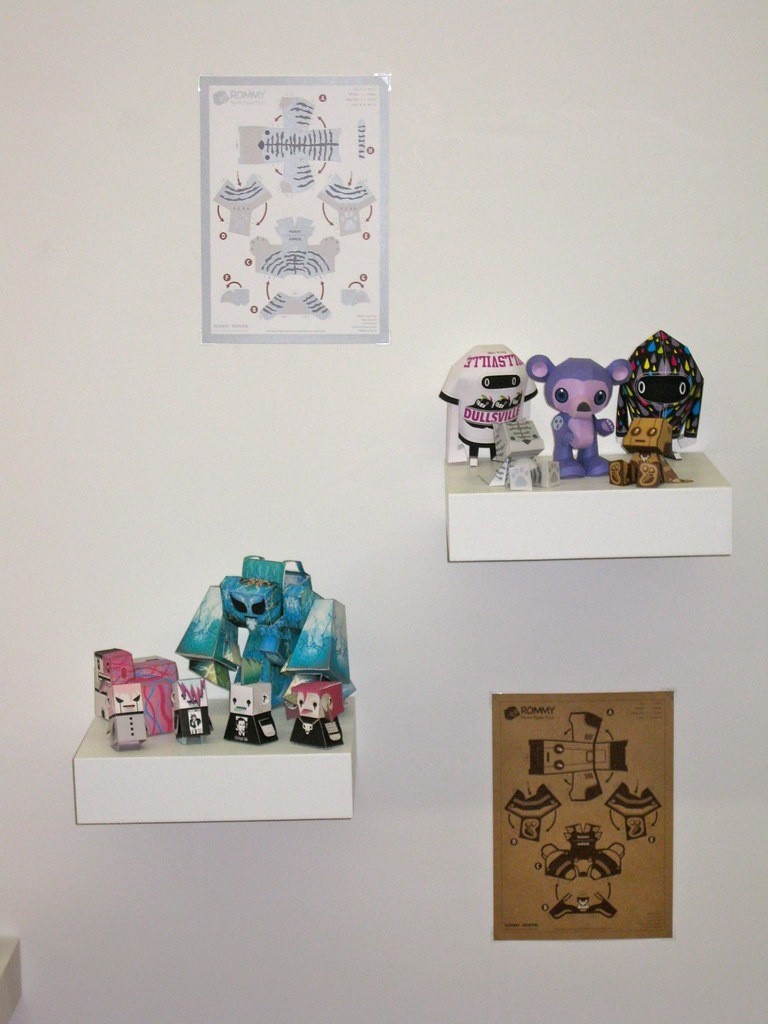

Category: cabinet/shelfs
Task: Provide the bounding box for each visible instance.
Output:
[72,454,731,825]
